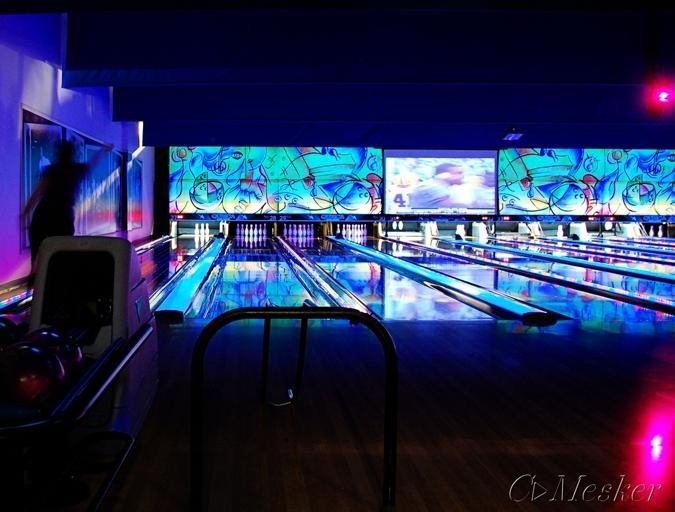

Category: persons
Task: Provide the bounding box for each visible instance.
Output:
[18,138,115,282]
[402,162,496,210]
